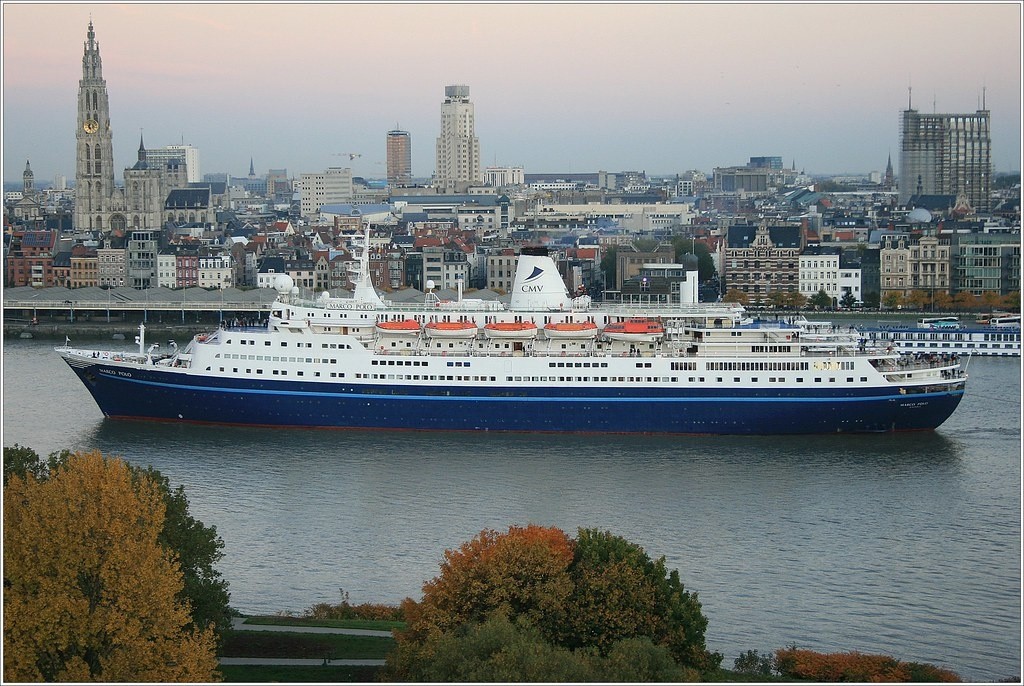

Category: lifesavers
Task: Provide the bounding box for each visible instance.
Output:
[436,303,439,307]
[105,352,108,356]
[501,352,506,356]
[442,351,445,356]
[561,351,566,356]
[827,330,831,334]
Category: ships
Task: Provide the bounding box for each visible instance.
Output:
[53,223,973,436]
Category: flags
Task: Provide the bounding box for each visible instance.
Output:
[66,336,71,342]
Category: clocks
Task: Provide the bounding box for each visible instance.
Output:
[84,120,99,134]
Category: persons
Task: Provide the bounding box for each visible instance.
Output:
[788,317,795,325]
[899,351,925,367]
[955,323,960,330]
[859,345,865,352]
[258,317,269,330]
[930,354,961,369]
[815,325,818,334]
[860,338,868,346]
[960,323,963,329]
[775,313,778,321]
[964,323,967,329]
[943,368,960,380]
[850,323,854,329]
[860,323,864,330]
[221,315,254,329]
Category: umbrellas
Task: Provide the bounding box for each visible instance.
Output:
[832,325,836,333]
[837,324,839,333]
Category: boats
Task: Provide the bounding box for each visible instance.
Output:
[422,320,479,339]
[603,317,665,343]
[544,320,599,341]
[483,321,540,339]
[374,318,421,333]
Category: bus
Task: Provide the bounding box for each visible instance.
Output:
[916,316,963,330]
[990,316,1021,329]
[976,312,1013,325]
